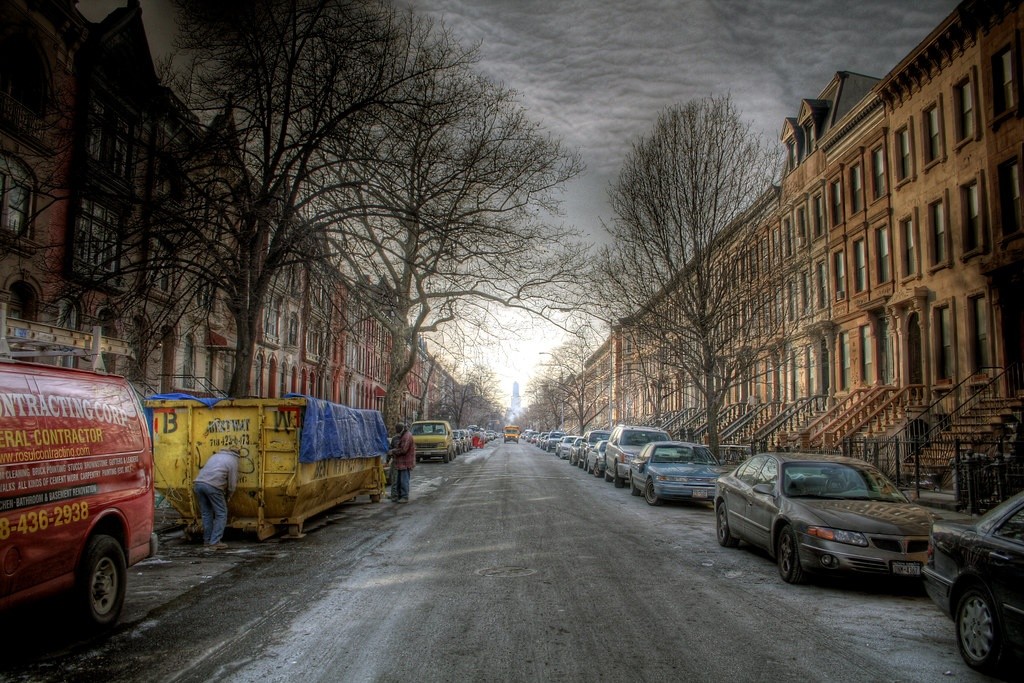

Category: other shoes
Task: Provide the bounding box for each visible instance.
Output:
[210,543,230,549]
[398,498,408,503]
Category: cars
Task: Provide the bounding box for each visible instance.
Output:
[712,452,943,592]
[630,440,729,506]
[521,429,565,455]
[568,437,584,466]
[919,491,1024,677]
[559,435,582,459]
[452,424,503,455]
[586,440,609,478]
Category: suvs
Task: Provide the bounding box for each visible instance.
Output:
[409,420,457,463]
[604,424,678,489]
[577,430,612,471]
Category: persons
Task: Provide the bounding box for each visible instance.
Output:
[191,445,241,550]
[388,422,415,505]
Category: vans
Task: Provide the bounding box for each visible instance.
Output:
[0,356,159,640]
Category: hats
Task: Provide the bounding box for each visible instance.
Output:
[395,424,406,432]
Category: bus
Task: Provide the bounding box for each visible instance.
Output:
[501,425,523,445]
